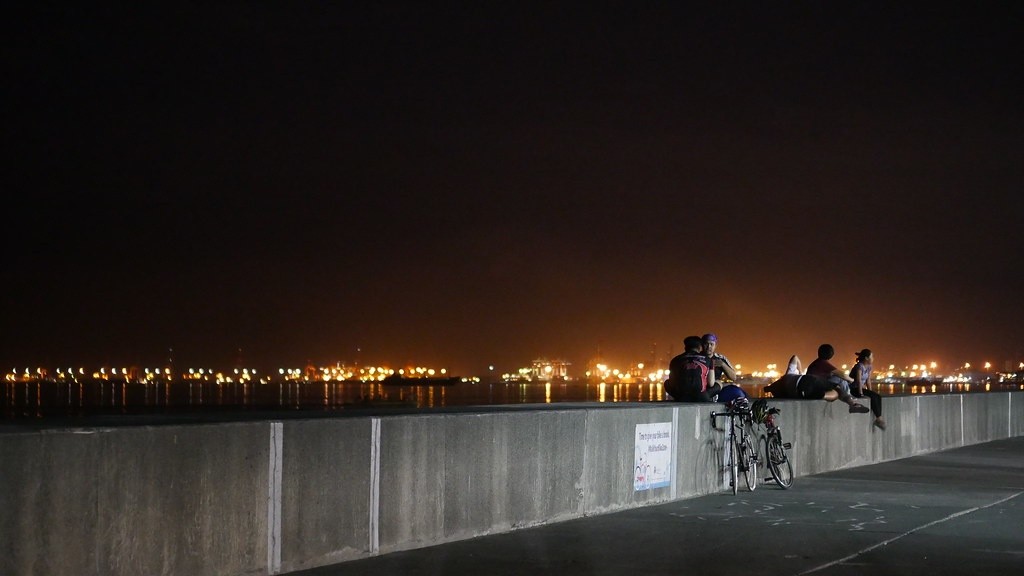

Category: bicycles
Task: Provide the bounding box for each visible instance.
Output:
[710,397,795,494]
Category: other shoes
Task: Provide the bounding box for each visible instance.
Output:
[874,420,890,431]
[849,403,869,413]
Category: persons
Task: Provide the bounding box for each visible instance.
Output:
[700,335,744,403]
[806,344,857,400]
[664,336,722,401]
[763,356,870,414]
[849,349,886,430]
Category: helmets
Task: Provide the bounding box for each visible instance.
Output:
[750,399,769,424]
[726,397,749,412]
